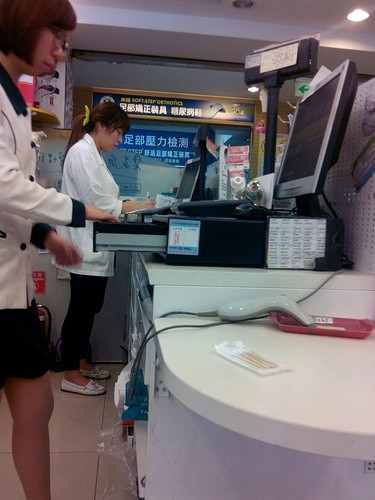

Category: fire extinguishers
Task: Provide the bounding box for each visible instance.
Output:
[36,300,52,346]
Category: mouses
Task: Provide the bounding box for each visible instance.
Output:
[233,204,276,220]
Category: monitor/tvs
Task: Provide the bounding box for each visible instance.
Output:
[174,157,200,202]
[272,59,358,216]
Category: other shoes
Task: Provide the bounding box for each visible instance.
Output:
[80,366,111,379]
[61,377,105,396]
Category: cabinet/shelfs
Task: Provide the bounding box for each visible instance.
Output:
[122,250,375,500]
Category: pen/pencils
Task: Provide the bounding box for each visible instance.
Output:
[147,192,151,199]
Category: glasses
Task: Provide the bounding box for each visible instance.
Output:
[49,25,71,55]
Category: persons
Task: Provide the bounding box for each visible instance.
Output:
[53,104,156,395]
[0,0,76,500]
